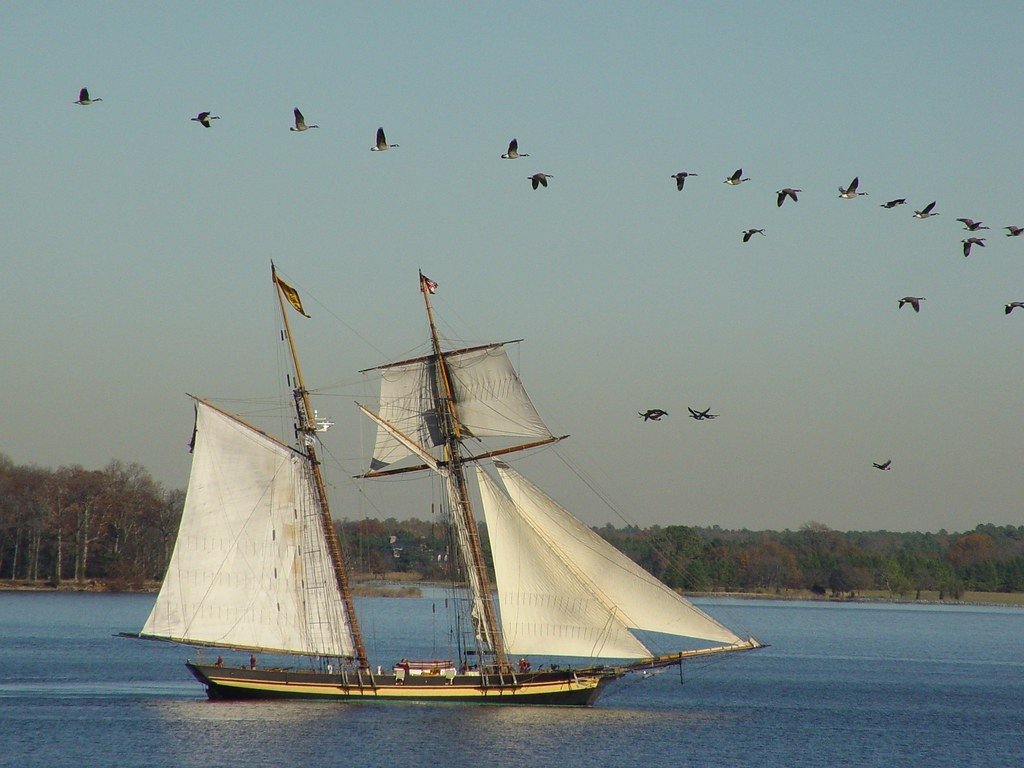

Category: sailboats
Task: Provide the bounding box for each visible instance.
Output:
[114,260,771,704]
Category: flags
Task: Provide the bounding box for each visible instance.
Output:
[272,273,311,318]
[420,275,438,294]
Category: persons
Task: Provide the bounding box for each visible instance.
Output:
[520,659,532,672]
[250,655,256,670]
[218,656,223,668]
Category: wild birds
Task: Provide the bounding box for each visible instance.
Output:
[912,200,940,219]
[71,86,102,106]
[1002,225,1024,238]
[370,126,399,151]
[742,228,766,242]
[527,172,554,191]
[723,168,751,186]
[956,218,991,232]
[501,138,531,159]
[190,112,222,127]
[872,459,892,471]
[1004,301,1024,315]
[879,197,908,208]
[898,296,927,313]
[776,188,803,207]
[290,107,320,132]
[670,171,699,191]
[960,237,987,258]
[639,404,717,422]
[838,176,869,199]
